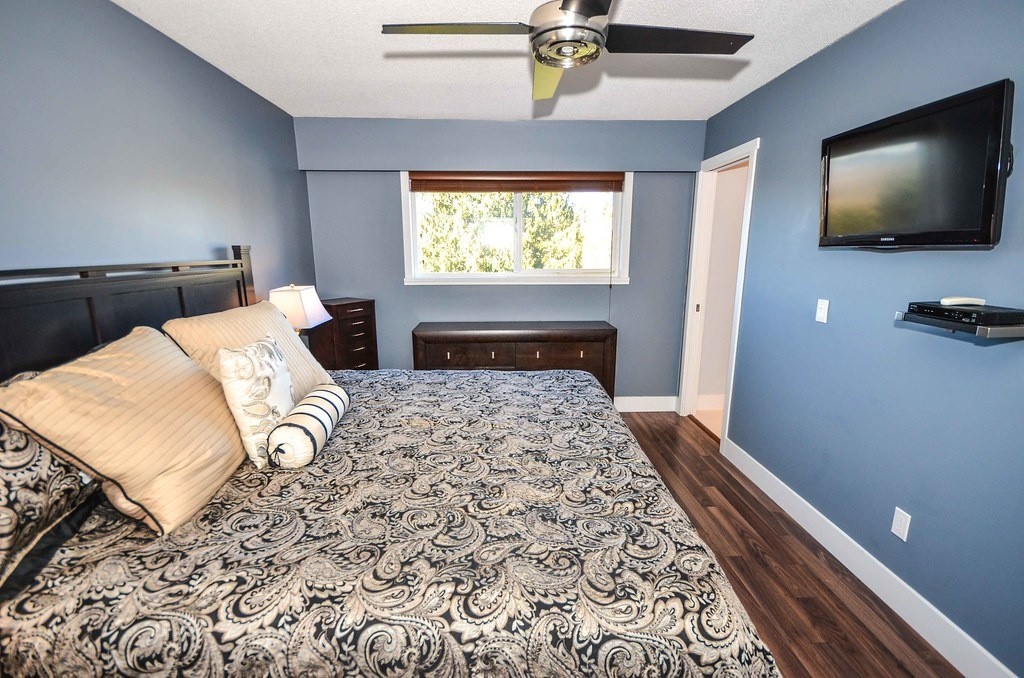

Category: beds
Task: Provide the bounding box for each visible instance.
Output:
[0,245,783,678]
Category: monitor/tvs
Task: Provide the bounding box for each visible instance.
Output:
[819,78,1015,247]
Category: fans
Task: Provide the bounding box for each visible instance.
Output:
[382,0,755,100]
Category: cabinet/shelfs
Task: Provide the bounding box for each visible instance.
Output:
[308,296,379,370]
[412,321,618,405]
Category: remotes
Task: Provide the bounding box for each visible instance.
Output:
[940,297,985,305]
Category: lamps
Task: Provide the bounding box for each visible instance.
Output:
[269,283,333,335]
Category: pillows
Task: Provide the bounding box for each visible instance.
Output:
[267,383,351,469]
[0,369,103,589]
[0,326,247,538]
[218,334,295,470]
[161,300,337,406]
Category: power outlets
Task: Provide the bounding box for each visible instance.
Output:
[891,506,912,542]
